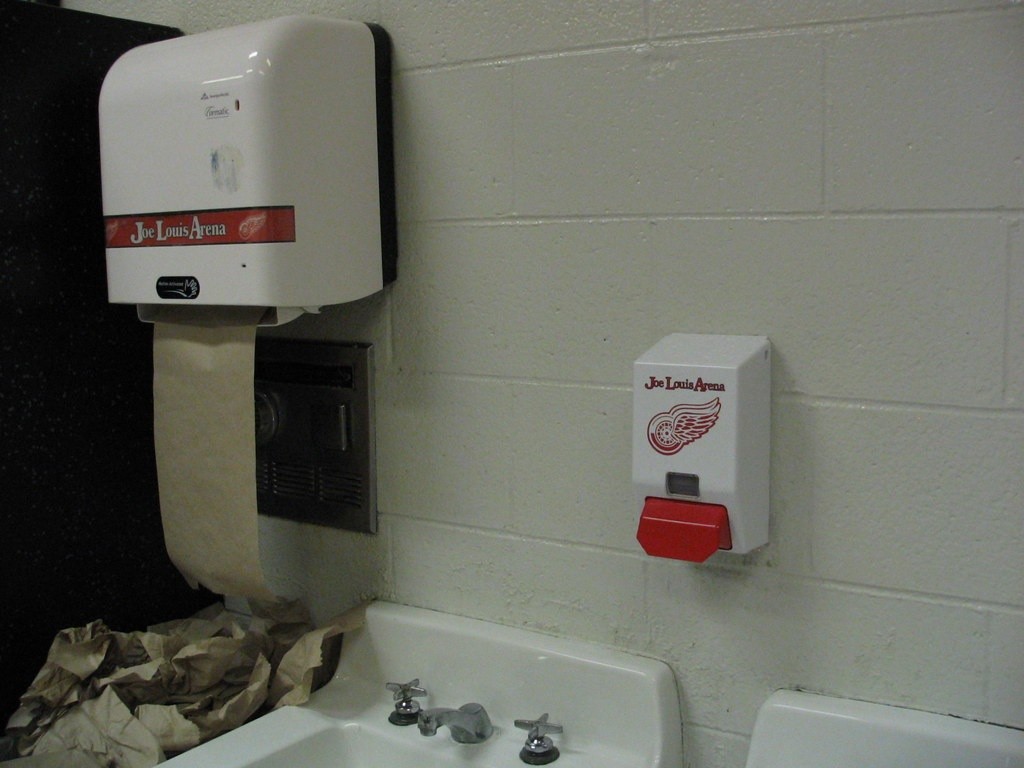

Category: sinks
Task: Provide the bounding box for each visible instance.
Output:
[149,598,689,766]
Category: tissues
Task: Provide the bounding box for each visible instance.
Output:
[93,13,401,608]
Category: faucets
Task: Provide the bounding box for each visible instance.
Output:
[416,702,494,745]
